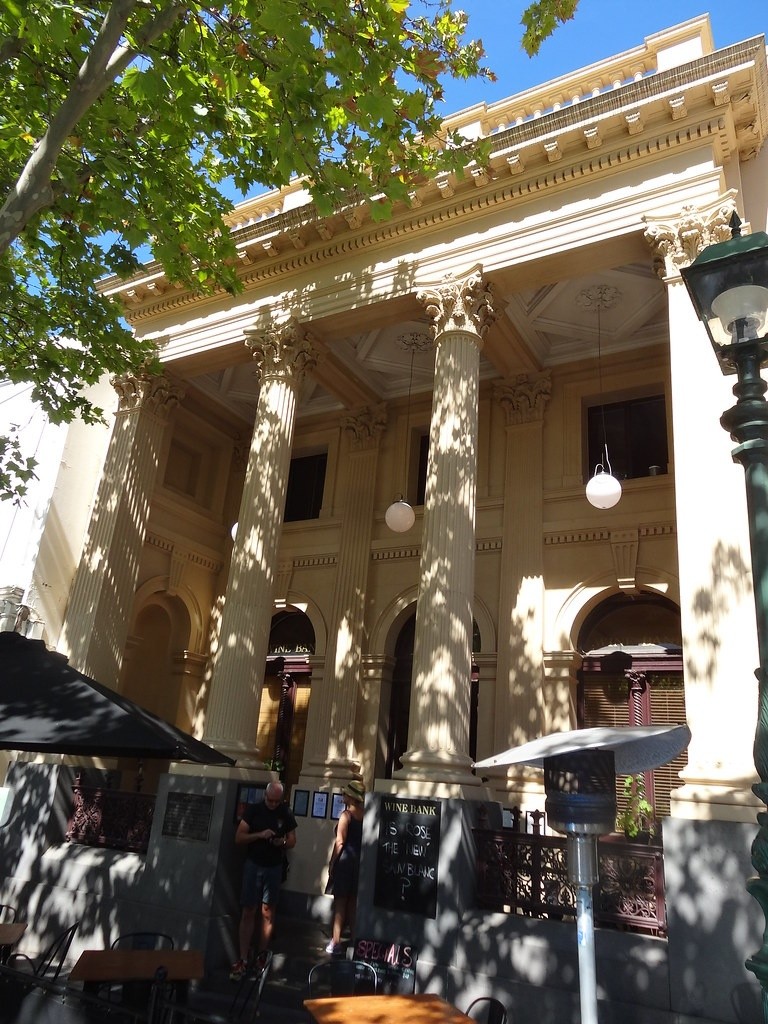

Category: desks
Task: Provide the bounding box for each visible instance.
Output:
[304,993,480,1024]
[64,949,204,1024]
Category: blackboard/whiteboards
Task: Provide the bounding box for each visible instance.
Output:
[347,937,418,996]
[372,795,442,920]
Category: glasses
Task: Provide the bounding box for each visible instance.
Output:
[266,794,281,804]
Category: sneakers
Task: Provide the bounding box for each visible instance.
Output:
[230,960,247,981]
[249,959,265,981]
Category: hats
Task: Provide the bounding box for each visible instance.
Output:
[340,780,365,802]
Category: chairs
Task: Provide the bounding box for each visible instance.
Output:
[159,949,273,1024]
[0,923,28,966]
[308,960,378,1024]
[3,922,79,995]
[86,932,174,1024]
[467,998,508,1024]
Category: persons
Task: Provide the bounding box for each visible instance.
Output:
[228,780,299,980]
[325,780,365,955]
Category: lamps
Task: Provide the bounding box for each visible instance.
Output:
[385,343,416,532]
[586,300,622,510]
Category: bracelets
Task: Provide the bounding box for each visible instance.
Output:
[281,840,287,847]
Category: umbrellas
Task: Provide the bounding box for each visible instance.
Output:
[0,632,238,768]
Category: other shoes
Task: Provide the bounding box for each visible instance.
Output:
[326,941,342,955]
